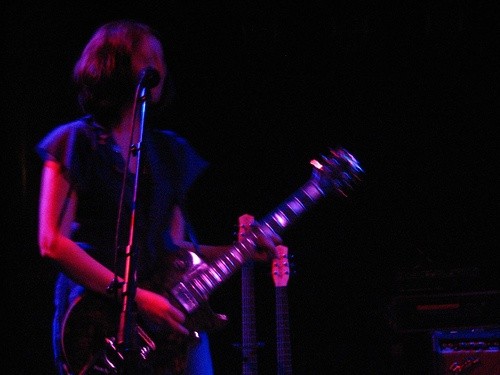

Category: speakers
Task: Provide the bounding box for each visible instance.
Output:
[431,328,500,375]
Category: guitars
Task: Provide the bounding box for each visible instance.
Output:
[61,146,362,375]
[269,243,292,369]
[234,211,262,371]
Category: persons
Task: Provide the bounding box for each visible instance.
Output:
[36,19,282,375]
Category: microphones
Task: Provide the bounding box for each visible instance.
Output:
[138,66,161,89]
